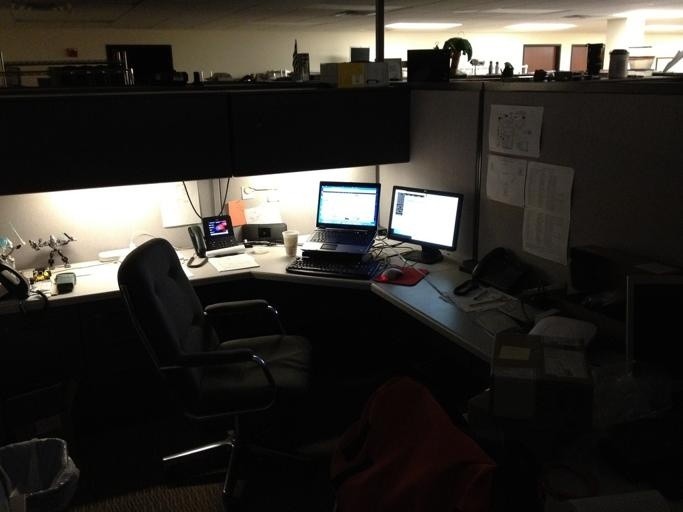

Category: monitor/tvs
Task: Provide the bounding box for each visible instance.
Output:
[625,275,682,381]
[387,186,463,265]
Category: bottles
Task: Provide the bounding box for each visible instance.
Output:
[488,60,493,75]
[495,61,499,74]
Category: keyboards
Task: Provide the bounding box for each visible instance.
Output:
[286,250,381,280]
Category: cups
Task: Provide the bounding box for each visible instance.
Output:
[200,70,213,90]
[282,230,299,258]
[608,49,630,78]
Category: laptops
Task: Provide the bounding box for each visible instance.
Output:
[300,182,380,254]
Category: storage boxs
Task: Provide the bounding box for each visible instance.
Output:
[319,62,367,88]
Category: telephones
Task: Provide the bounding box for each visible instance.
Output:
[0,264,31,301]
[471,247,526,295]
[188,215,238,258]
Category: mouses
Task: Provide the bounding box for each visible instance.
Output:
[381,267,404,281]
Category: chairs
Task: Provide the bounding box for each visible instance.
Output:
[118,239,319,512]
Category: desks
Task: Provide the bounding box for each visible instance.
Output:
[0,240,683,477]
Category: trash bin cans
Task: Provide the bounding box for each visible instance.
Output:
[0,438,72,512]
